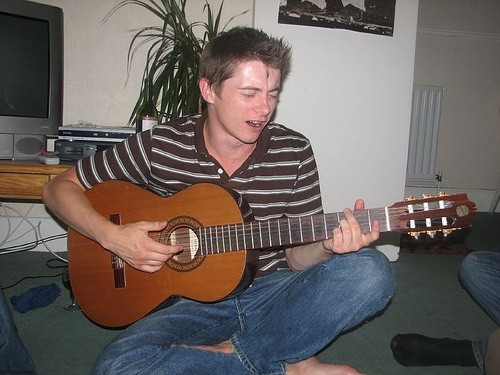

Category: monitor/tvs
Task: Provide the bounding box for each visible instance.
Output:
[0,0,64,137]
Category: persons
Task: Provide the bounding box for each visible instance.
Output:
[392,251,499,375]
[41,27,396,375]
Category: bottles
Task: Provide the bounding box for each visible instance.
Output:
[137,78,157,134]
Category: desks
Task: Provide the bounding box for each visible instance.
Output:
[0,158,84,200]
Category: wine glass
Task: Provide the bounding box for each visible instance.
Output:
[63,267,82,313]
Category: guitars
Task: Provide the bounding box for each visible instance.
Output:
[66,177,478,330]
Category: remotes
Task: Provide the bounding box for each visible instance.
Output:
[38,155,60,165]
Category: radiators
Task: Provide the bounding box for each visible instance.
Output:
[405,84,446,188]
